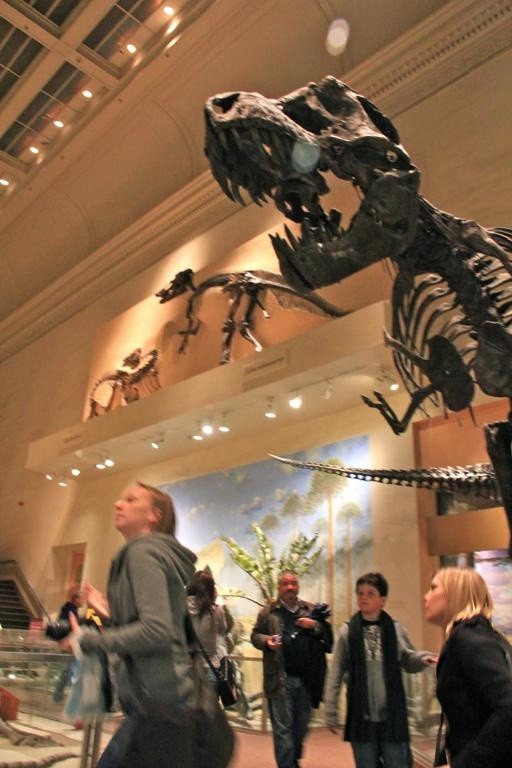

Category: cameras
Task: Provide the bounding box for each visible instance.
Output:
[46,601,101,640]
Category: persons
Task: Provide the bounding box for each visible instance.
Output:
[44,481,240,766]
[250,567,335,767]
[179,561,238,725]
[323,572,440,768]
[425,566,510,767]
[47,579,84,731]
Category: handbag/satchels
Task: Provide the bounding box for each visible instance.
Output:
[218,655,237,706]
[63,627,106,718]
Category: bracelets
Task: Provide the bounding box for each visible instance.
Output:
[69,625,92,657]
